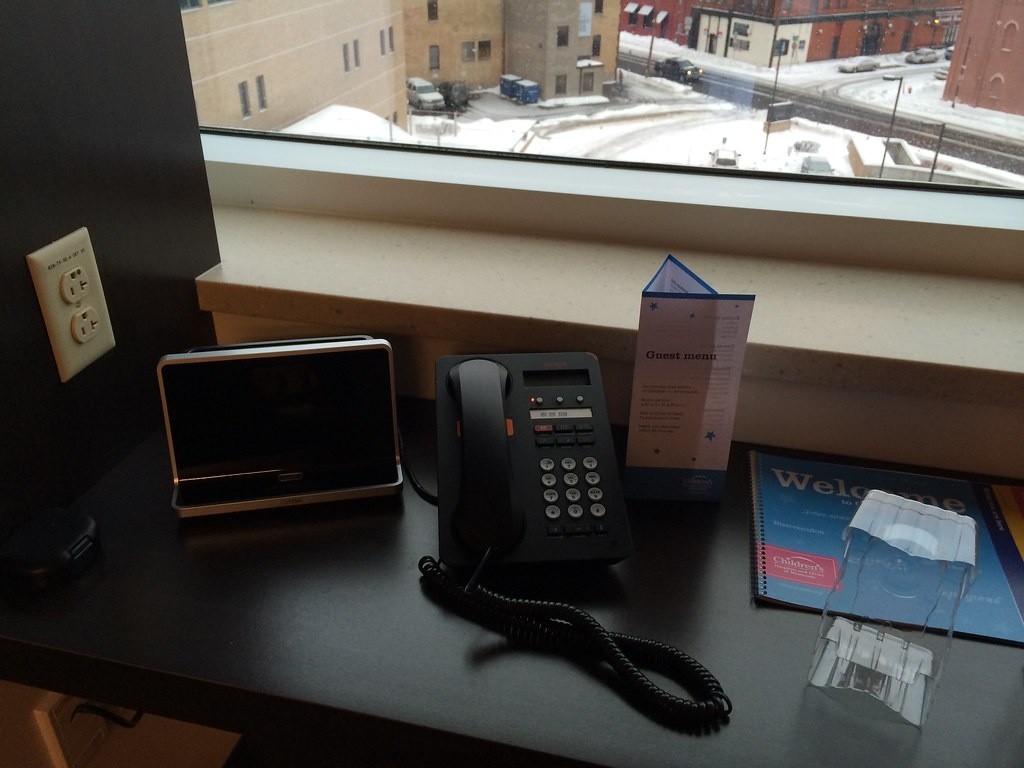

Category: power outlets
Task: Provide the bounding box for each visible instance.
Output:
[26,227,116,383]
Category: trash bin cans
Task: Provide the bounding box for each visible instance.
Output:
[499,73,538,103]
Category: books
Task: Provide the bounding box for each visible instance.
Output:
[744,448,1024,646]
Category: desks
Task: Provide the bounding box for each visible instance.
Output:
[0,391,1024,768]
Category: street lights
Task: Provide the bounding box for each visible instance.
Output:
[879,74,904,178]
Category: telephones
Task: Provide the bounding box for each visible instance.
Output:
[435,351,630,567]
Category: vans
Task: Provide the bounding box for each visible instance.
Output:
[406,77,445,110]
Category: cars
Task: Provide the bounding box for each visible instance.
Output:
[935,66,952,80]
[801,155,835,177]
[839,56,880,73]
[709,149,742,170]
[905,47,938,63]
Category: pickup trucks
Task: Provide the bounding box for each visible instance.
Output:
[656,56,701,83]
